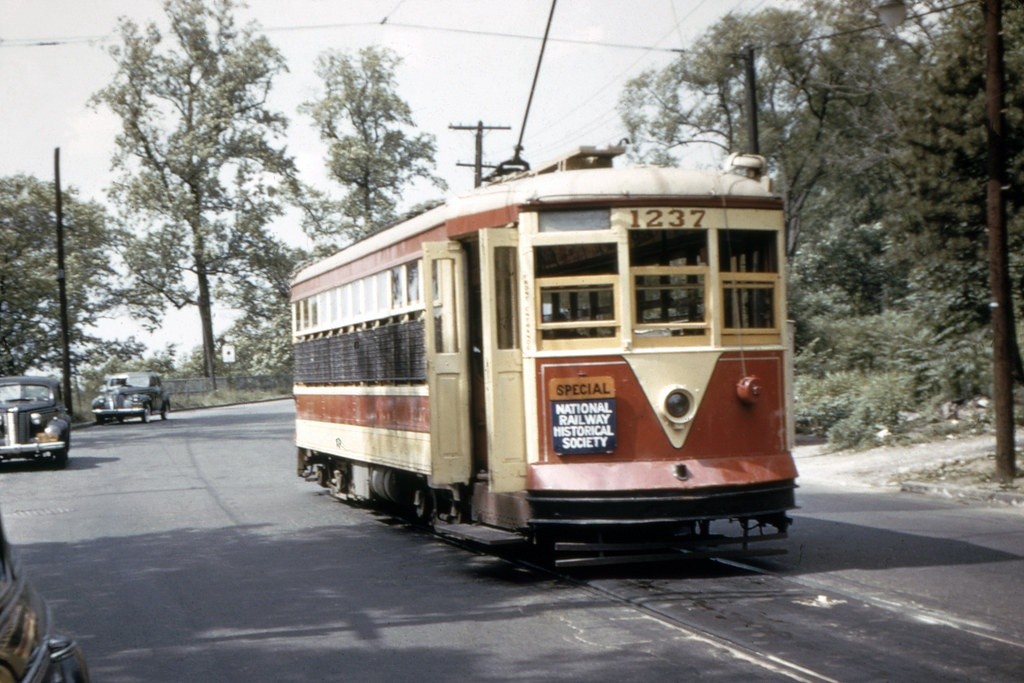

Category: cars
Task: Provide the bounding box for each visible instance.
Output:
[92,372,171,423]
[0,375,73,470]
[0,511,91,683]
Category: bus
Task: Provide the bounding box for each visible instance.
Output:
[288,1,801,568]
